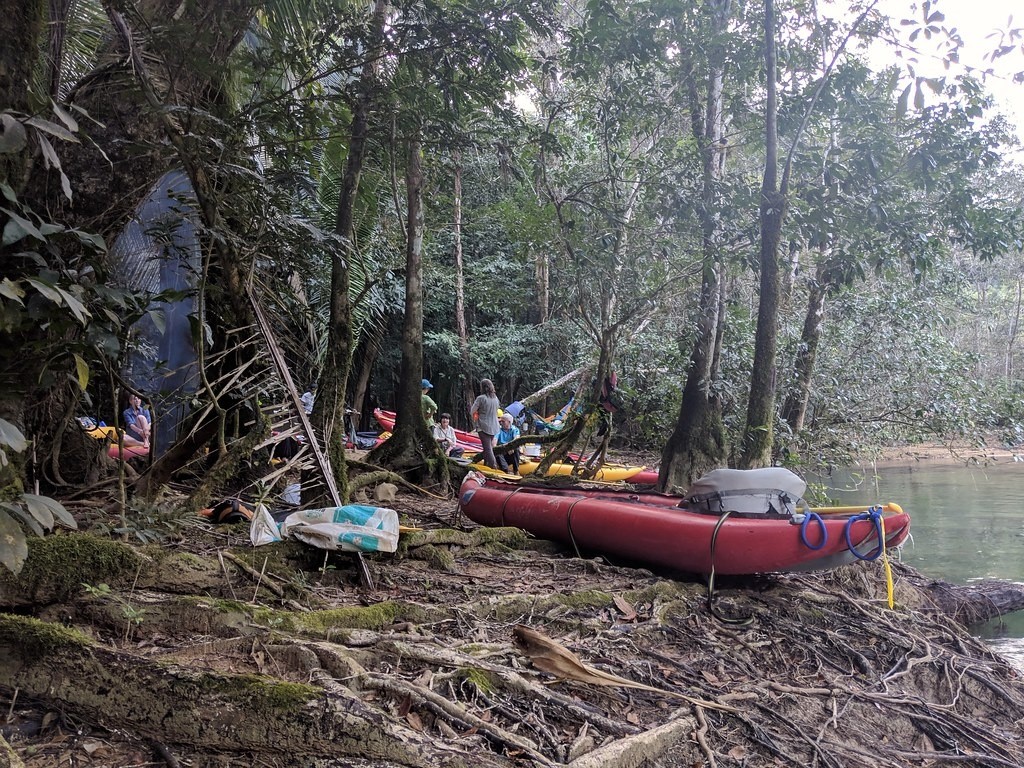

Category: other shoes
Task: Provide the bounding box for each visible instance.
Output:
[504,470,511,474]
[513,469,520,475]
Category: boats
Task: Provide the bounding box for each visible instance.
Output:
[85,425,150,460]
[460,471,911,578]
[377,436,660,483]
[373,408,609,469]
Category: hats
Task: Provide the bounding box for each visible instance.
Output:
[310,384,318,390]
[422,379,433,388]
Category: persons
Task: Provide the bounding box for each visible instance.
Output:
[301,383,318,421]
[118,394,151,449]
[491,413,520,477]
[433,413,465,458]
[421,379,438,435]
[471,379,500,469]
